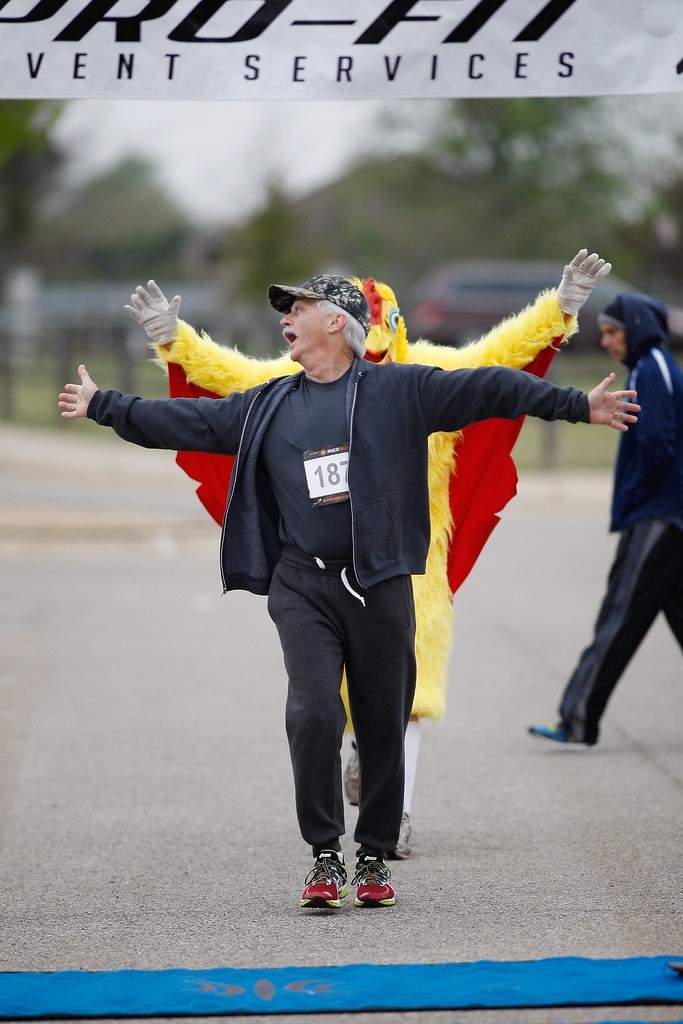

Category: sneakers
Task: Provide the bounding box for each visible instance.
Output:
[525,723,597,753]
[351,851,396,907]
[342,737,363,805]
[298,849,349,909]
[384,812,413,860]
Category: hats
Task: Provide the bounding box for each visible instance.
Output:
[266,273,371,339]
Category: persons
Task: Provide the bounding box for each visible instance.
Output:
[121,246,612,858]
[530,294,682,749]
[53,276,645,915]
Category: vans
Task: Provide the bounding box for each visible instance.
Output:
[412,260,683,355]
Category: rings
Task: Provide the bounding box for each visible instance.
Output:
[614,411,618,419]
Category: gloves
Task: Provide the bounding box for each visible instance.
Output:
[124,276,182,346]
[556,248,611,318]
